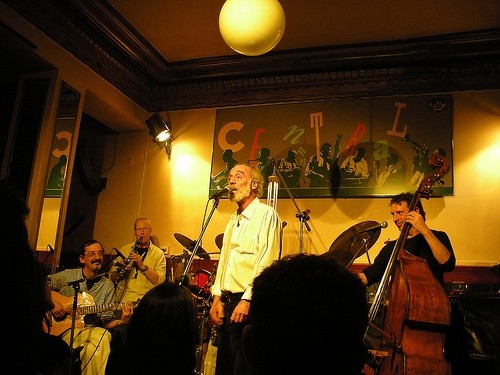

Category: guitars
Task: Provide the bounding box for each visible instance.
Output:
[41,290,140,337]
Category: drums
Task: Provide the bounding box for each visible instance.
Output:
[366,297,389,339]
[189,269,212,295]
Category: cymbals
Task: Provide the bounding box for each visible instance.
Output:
[174,232,210,253]
[214,233,224,250]
[328,220,381,262]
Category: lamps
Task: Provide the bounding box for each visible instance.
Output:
[144,112,172,156]
[219,0,286,56]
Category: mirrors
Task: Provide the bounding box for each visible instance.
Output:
[35,81,81,276]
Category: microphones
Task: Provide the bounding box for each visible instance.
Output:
[210,186,233,199]
[359,221,388,233]
[114,261,126,268]
[49,244,54,255]
[114,249,127,261]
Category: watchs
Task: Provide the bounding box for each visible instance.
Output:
[142,266,148,272]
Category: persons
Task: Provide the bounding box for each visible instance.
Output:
[105,282,198,375]
[358,193,456,375]
[110,216,167,308]
[42,239,133,375]
[245,253,368,375]
[209,164,283,375]
[0,194,80,375]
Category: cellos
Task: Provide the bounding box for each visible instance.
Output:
[358,147,452,375]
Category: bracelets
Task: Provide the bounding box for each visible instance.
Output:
[244,299,251,302]
